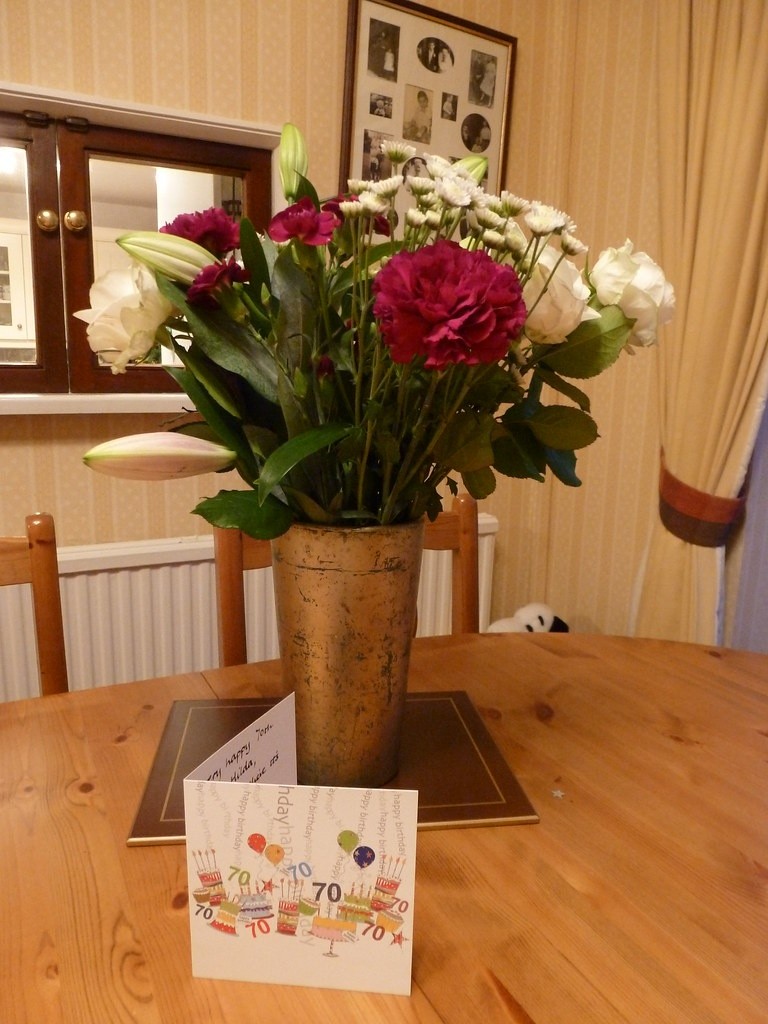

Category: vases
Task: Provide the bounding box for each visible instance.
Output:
[269,512,421,789]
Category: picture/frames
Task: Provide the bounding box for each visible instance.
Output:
[338,1,520,194]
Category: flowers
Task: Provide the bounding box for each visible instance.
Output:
[73,121,675,552]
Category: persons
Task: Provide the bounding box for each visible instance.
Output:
[373,100,392,117]
[423,39,452,73]
[410,90,432,138]
[473,53,496,108]
[370,143,386,181]
[371,31,395,79]
[462,121,491,152]
[442,94,455,119]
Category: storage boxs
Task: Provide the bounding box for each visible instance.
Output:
[0,217,140,350]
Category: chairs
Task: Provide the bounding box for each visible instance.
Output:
[0,512,72,696]
[212,489,480,667]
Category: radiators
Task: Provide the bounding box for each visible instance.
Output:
[1,511,497,706]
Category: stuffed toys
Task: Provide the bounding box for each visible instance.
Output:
[486,604,568,635]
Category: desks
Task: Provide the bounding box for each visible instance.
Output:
[0,631,768,1024]
